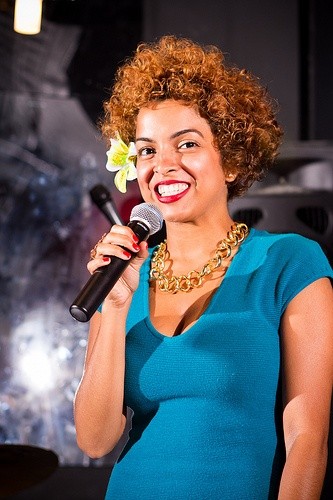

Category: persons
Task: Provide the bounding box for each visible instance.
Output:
[73,36,333,500]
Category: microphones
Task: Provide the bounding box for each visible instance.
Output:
[88,183,124,226]
[69,201,164,322]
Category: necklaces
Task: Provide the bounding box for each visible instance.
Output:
[149,221,248,293]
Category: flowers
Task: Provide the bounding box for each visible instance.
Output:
[106,131,138,193]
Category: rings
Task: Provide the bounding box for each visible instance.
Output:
[89,233,108,260]
